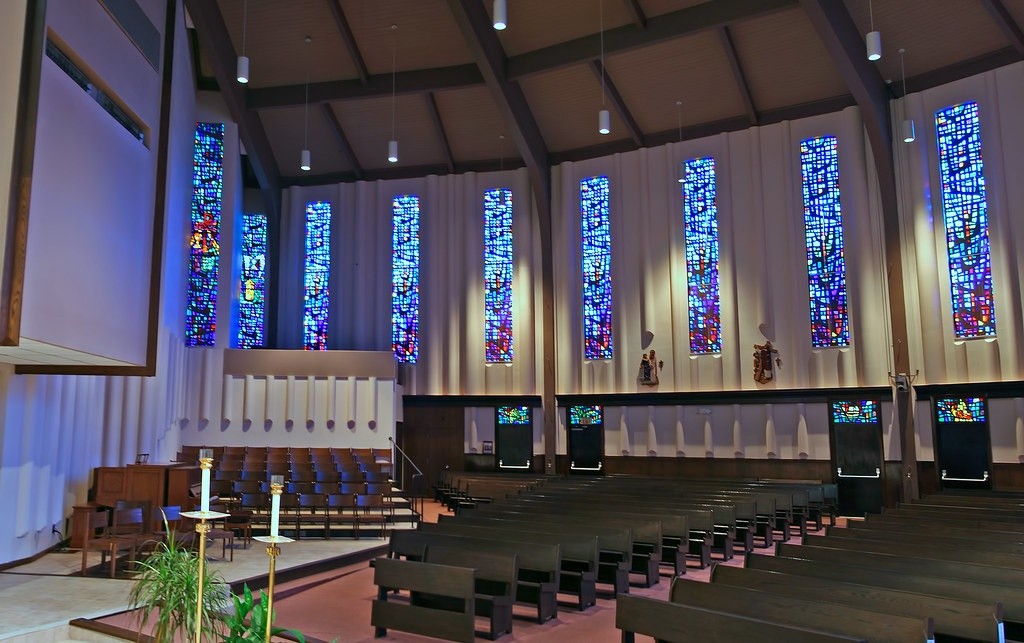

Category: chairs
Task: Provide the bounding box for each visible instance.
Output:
[81,442,393,579]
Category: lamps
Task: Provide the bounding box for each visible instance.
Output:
[301,37,311,170]
[388,24,399,162]
[599,0,610,134]
[493,0,507,30]
[866,0,882,61]
[899,48,916,143]
[237,0,249,83]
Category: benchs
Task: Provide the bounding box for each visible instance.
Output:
[367,470,1023,642]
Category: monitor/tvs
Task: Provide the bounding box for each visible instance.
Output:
[934,397,989,427]
[568,403,603,427]
[497,404,532,425]
[832,400,880,425]
